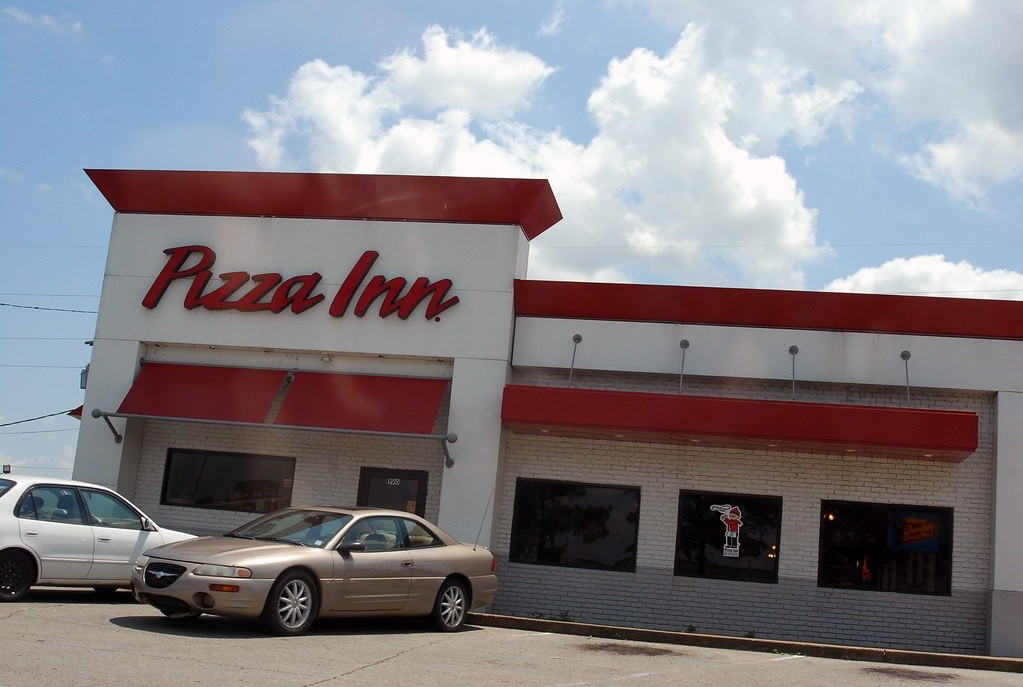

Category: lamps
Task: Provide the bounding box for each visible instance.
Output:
[789,346,798,400]
[823,506,835,521]
[320,355,332,361]
[568,334,582,386]
[901,350,910,407]
[679,338,689,393]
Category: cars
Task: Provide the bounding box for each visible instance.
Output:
[130,503,497,636]
[0,473,200,603]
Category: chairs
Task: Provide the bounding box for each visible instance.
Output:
[58,494,83,523]
[23,496,50,519]
[361,533,394,550]
